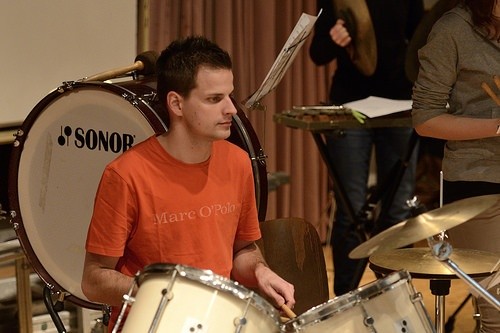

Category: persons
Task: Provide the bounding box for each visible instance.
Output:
[308,0,500,333]
[81,35,295,333]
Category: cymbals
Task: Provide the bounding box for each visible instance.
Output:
[347,193,500,260]
[369,244,500,275]
[403,0,460,85]
[333,0,378,78]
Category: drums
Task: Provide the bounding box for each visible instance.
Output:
[4,75,271,314]
[284,268,438,333]
[111,260,283,333]
